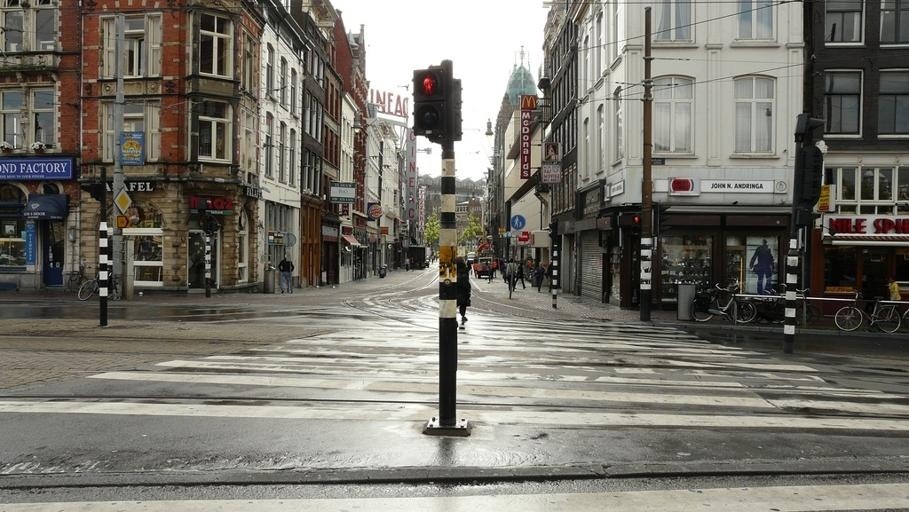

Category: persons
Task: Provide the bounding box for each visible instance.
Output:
[498,257,553,293]
[749,239,776,294]
[277,254,295,293]
[455,256,472,321]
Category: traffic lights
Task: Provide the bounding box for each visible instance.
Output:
[412,67,445,138]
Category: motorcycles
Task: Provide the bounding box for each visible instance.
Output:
[378,264,387,278]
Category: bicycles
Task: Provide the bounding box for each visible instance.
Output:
[68,261,121,301]
[690,283,821,329]
[835,287,901,334]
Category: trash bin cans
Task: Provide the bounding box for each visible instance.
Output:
[265,270,275,293]
[379,268,385,278]
[678,286,696,321]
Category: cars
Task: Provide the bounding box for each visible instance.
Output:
[467,252,495,279]
